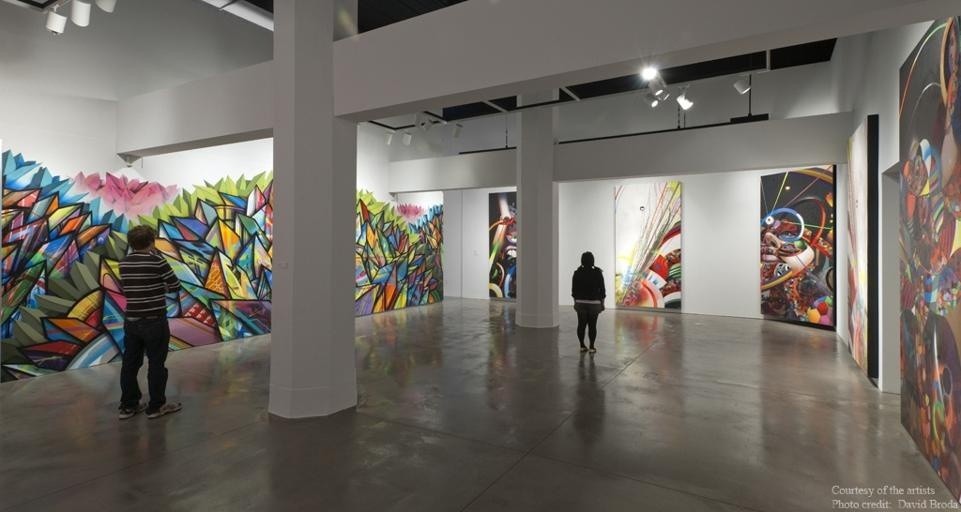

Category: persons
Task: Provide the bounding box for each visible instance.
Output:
[572,251,606,353]
[117,225,182,419]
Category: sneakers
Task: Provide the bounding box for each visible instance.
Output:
[118,398,184,419]
[580,347,596,353]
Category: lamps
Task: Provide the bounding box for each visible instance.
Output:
[43,0,117,37]
[641,77,671,108]
[676,74,752,112]
